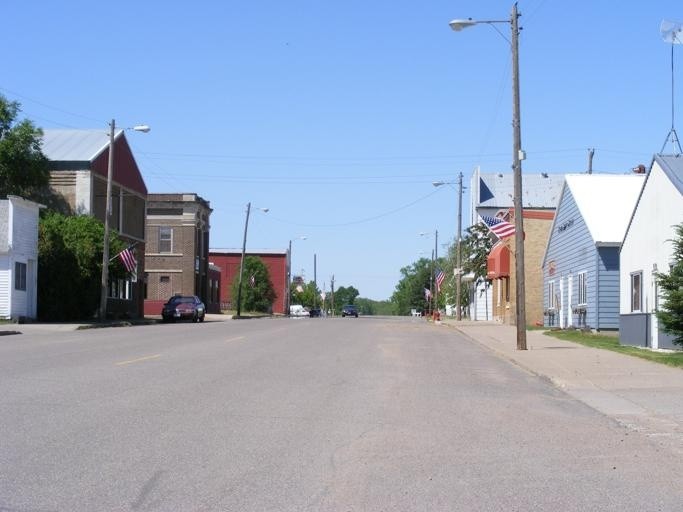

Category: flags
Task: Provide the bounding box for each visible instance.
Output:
[120,245,137,272]
[434,268,445,291]
[481,215,525,240]
[247,275,255,288]
[424,288,430,301]
[296,286,303,294]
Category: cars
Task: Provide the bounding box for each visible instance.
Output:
[310,309,321,317]
[161,295,206,322]
[342,305,359,318]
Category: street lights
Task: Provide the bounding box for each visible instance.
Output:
[431,172,463,321]
[95,119,151,320]
[419,230,437,259]
[450,1,526,349]
[287,236,307,316]
[235,202,269,314]
